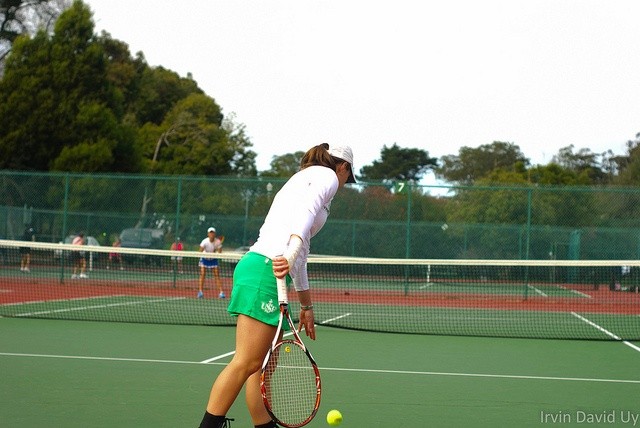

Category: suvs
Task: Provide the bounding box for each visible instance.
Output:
[112,228,164,267]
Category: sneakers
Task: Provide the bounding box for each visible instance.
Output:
[79,273,89,279]
[71,273,78,280]
[24,267,31,274]
[20,267,24,272]
[197,291,203,298]
[218,293,225,298]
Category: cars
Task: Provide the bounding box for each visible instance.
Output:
[54,235,101,259]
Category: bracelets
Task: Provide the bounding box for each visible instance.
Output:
[299,303,313,311]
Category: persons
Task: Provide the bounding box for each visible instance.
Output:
[70,234,90,280]
[19,228,36,272]
[198,141,357,425]
[108,240,124,272]
[170,237,183,273]
[199,226,225,298]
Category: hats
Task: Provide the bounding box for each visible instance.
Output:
[326,143,358,184]
[208,227,216,233]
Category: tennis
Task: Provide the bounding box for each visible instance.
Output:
[326,409,342,426]
